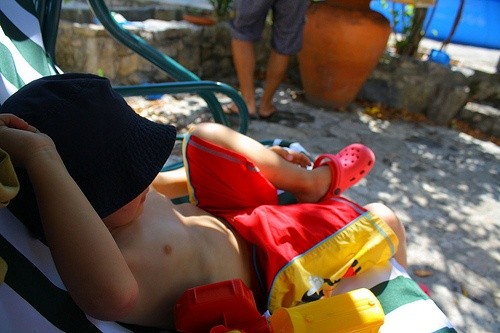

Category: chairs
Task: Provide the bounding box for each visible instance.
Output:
[0,0,458,333]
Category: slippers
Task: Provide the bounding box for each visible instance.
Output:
[258,106,277,119]
[311,144,375,204]
[222,104,257,120]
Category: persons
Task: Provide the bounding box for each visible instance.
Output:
[220,0,315,118]
[0,73,407,328]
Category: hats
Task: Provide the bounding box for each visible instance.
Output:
[0,73,176,226]
